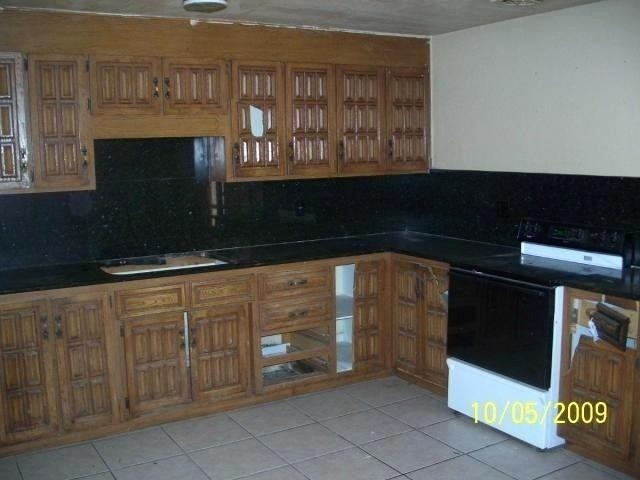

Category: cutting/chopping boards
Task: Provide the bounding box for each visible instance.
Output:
[100,252,227,276]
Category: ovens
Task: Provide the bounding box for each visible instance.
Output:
[444,267,553,453]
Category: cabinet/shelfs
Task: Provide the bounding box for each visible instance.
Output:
[0,269,252,447]
[233,26,429,177]
[557,292,638,472]
[0,11,98,192]
[257,253,450,395]
[100,14,230,140]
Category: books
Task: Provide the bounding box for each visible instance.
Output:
[260,335,290,357]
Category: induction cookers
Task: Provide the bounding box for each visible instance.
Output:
[454,250,597,287]
